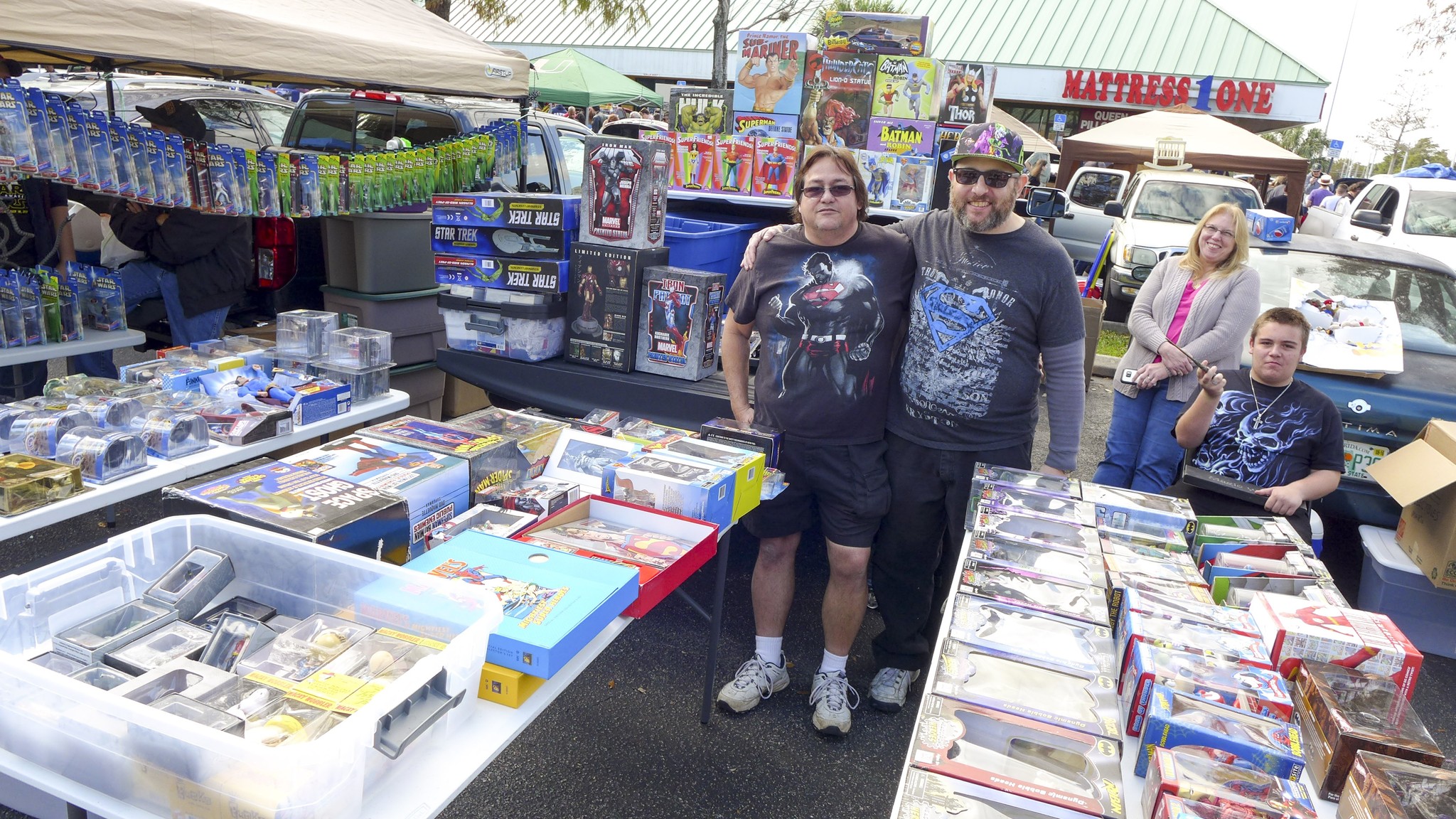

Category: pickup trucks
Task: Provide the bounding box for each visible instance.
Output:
[1299,174,1456,272]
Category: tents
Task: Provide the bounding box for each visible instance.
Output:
[0,0,531,192]
[528,48,664,128]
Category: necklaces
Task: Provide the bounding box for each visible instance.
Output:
[1193,269,1201,290]
[1249,369,1293,430]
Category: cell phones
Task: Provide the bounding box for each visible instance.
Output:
[1119,368,1162,388]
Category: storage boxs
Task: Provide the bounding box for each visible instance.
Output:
[1,13,1456,819]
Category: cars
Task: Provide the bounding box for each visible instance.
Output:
[1243,233,1456,534]
[595,116,673,145]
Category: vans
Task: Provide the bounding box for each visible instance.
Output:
[265,85,598,312]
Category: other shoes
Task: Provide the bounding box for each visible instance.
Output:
[867,584,878,609]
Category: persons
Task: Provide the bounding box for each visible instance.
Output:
[1297,162,1369,231]
[1024,139,1053,222]
[1083,161,1107,168]
[0,59,255,404]
[529,98,669,134]
[1158,308,1346,547]
[717,143,917,740]
[1239,175,1287,215]
[739,120,1087,713]
[491,98,520,103]
[1090,203,1259,494]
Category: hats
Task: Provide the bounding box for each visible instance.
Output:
[951,122,1025,175]
[1311,163,1322,172]
[135,100,207,142]
[1317,175,1334,185]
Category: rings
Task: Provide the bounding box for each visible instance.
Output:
[1211,379,1217,385]
[1148,376,1153,381]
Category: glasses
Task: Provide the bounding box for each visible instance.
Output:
[953,167,1019,188]
[800,184,855,198]
[1203,223,1235,239]
[1312,171,1320,172]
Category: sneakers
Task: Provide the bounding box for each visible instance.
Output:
[809,663,860,736]
[868,668,921,714]
[715,650,790,714]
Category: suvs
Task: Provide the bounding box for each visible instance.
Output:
[1052,169,1266,325]
[6,69,297,187]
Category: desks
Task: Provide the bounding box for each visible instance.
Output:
[1,388,411,542]
[1,326,146,402]
[0,526,733,818]
